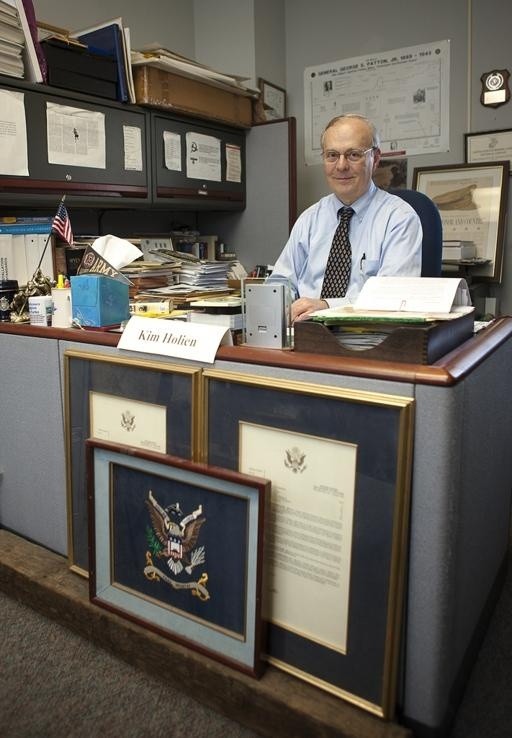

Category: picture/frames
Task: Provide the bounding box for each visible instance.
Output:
[411,161,510,286]
[464,125,512,163]
[61,346,206,582]
[81,436,272,682]
[195,366,418,725]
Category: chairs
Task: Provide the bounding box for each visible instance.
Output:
[386,187,443,279]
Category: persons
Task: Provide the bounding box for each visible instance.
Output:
[11,269,51,323]
[271,113,425,327]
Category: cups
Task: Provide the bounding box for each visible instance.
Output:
[49,286,74,328]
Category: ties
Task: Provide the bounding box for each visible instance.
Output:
[319,206,356,299]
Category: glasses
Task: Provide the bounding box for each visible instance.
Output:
[319,148,373,164]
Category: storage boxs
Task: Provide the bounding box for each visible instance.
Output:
[38,40,118,101]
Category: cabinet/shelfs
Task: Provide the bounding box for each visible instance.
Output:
[151,109,246,214]
[1,75,152,211]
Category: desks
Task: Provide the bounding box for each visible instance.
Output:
[0,311,512,730]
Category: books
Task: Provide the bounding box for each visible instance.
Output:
[121,260,181,297]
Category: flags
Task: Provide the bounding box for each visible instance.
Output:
[51,201,74,249]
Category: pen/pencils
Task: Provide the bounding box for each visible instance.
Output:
[56,274,70,288]
[251,267,262,277]
